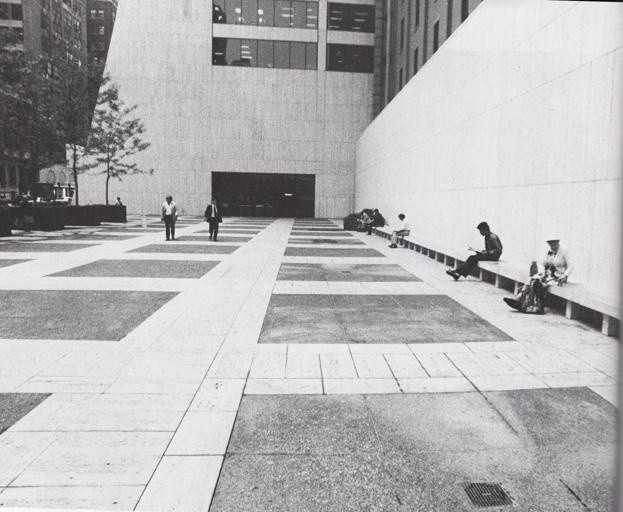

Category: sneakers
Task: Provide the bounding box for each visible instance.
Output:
[388,244,397,248]
[446,269,460,280]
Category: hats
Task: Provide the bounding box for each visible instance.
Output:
[545,233,560,242]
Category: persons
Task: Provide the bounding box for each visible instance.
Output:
[503,234,573,314]
[446,221,503,281]
[389,213,411,248]
[366,209,384,235]
[115,197,122,206]
[161,196,179,240]
[356,210,371,232]
[204,197,223,241]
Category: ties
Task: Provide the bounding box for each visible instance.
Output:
[214,205,216,217]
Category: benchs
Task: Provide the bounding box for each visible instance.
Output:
[370,224,620,337]
